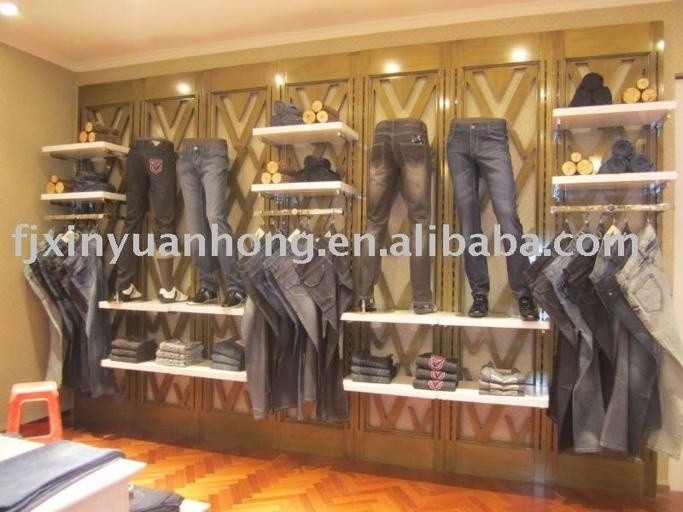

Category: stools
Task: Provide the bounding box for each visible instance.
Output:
[7,381,63,442]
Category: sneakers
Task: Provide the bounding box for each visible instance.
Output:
[519,297,539,321]
[469,295,489,317]
[221,291,246,307]
[122,284,143,301]
[159,286,188,302]
[359,295,376,312]
[414,302,437,313]
[189,287,217,304]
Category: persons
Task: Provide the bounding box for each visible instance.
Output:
[176,135,244,308]
[447,115,540,321]
[358,116,437,316]
[113,138,191,303]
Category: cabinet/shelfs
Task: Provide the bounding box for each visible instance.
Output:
[97,301,248,382]
[339,305,553,411]
[250,122,359,216]
[0,435,212,511]
[40,141,127,220]
[549,99,677,214]
